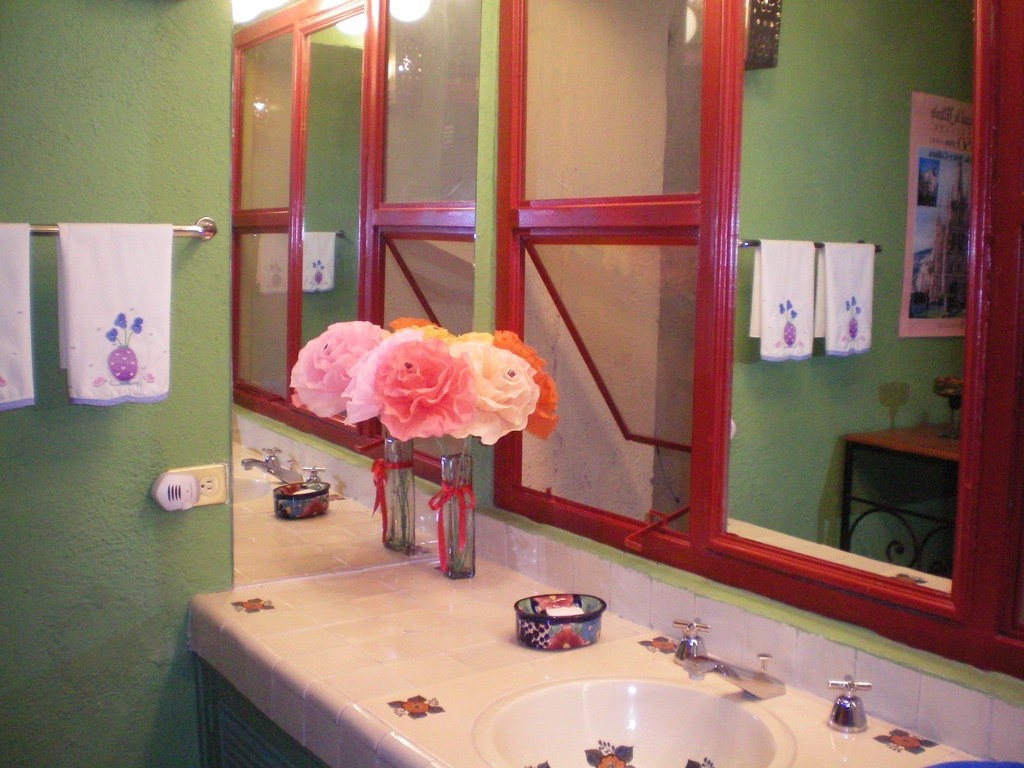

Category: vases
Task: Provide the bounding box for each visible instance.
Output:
[436,436,477,578]
[377,423,416,553]
[937,398,962,436]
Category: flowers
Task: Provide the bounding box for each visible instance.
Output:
[934,377,966,398]
[341,324,560,445]
[288,317,432,420]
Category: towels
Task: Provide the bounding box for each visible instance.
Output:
[749,238,817,362]
[0,222,35,411]
[57,222,173,406]
[813,240,876,359]
[302,231,336,294]
[256,233,288,296]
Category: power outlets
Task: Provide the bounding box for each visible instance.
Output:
[167,464,227,508]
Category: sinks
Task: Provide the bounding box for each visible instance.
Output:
[232,478,273,504]
[471,676,798,768]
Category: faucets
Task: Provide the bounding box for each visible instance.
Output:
[240,447,302,484]
[683,655,786,699]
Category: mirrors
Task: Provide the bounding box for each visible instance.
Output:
[229,1,483,587]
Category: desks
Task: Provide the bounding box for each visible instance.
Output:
[836,429,963,580]
